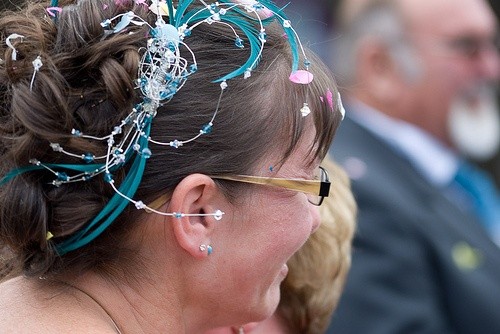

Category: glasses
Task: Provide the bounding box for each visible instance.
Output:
[146,166,331,214]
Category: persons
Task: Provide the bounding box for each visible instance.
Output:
[330,0,500,334]
[1,0,343,334]
[205,154,358,334]
